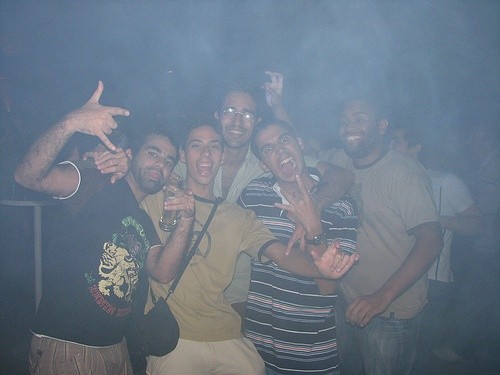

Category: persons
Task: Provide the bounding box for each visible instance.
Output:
[98,120,361,375]
[174,75,354,260]
[13,79,196,375]
[389,123,482,375]
[262,70,444,375]
[58,116,131,162]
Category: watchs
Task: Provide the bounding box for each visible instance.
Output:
[303,232,326,246]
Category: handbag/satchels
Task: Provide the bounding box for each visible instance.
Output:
[134,296,180,357]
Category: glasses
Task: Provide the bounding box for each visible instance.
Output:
[222,107,256,128]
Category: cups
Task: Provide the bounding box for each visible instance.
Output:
[158,180,186,231]
